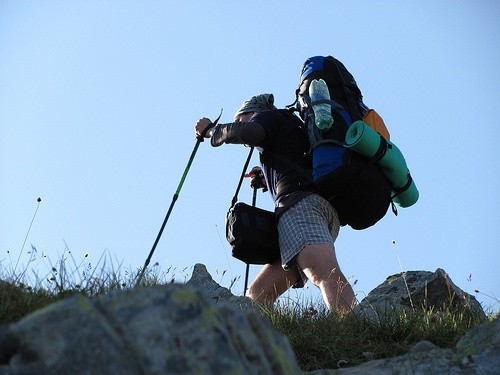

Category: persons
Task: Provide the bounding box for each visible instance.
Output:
[195,93,356,316]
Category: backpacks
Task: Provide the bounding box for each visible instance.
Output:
[288,54,396,230]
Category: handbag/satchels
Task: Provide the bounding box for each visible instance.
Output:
[225,202,280,267]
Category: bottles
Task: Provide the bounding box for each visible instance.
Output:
[309,79,333,129]
